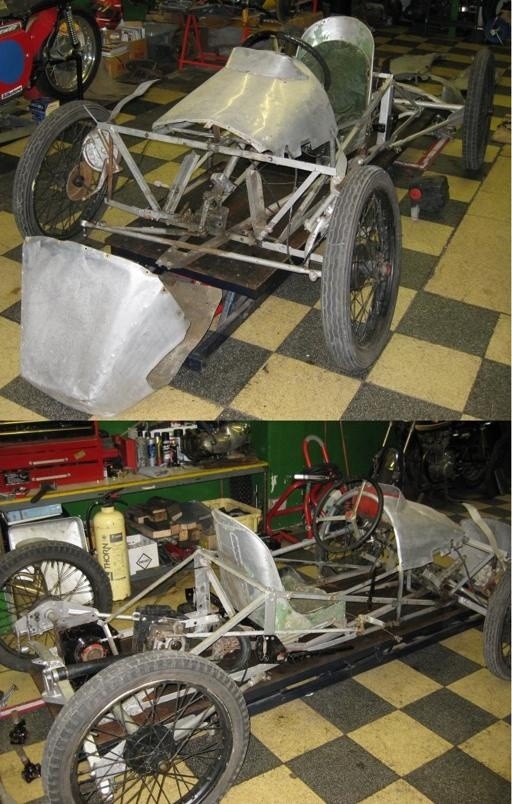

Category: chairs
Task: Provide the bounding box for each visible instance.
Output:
[208,508,347,660]
[289,13,377,155]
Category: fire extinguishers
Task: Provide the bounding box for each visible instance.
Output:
[86,487,131,601]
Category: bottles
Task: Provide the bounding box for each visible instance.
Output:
[133,430,186,467]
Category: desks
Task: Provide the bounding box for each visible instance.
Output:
[1,455,269,636]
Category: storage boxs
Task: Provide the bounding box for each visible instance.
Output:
[101,37,149,78]
[196,495,263,550]
